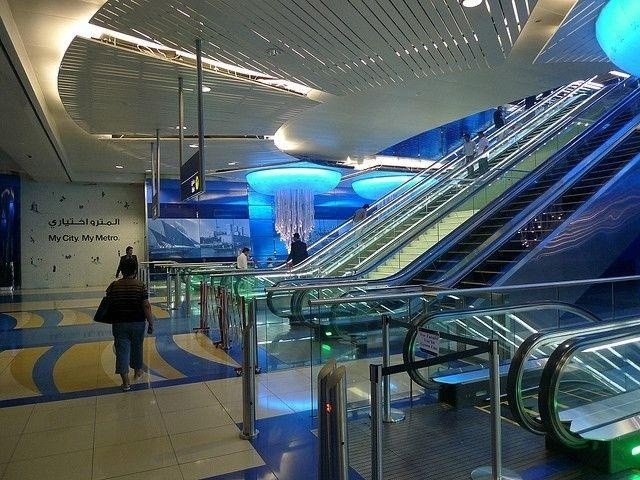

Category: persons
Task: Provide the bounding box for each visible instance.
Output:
[237,248,250,269]
[524,86,565,120]
[353,204,369,226]
[494,105,506,141]
[462,134,476,179]
[476,129,490,175]
[116,246,138,278]
[266,258,273,267]
[105,258,155,391]
[285,232,309,265]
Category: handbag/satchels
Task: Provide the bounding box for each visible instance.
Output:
[95,297,113,324]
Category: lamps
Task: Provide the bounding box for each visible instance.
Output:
[592,0,640,80]
[242,164,344,255]
[348,173,442,227]
